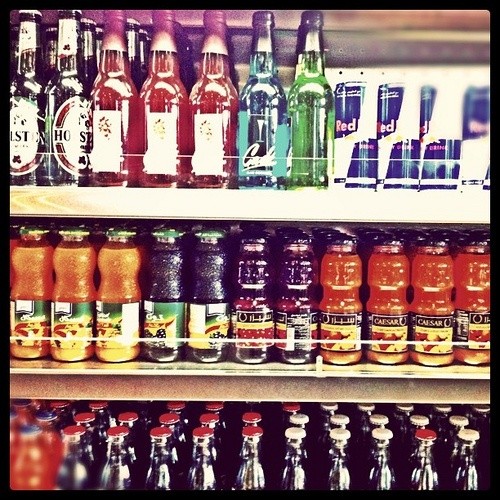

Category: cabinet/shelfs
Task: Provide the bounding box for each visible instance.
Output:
[10,185,492,405]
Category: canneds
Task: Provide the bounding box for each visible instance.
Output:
[332,82,490,190]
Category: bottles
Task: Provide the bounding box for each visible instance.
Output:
[232,237,275,363]
[453,242,491,364]
[286,13,334,191]
[141,8,191,187]
[192,13,236,188]
[97,232,142,361]
[321,238,363,365]
[50,229,97,360]
[410,239,456,366]
[365,241,409,364]
[92,11,140,182]
[275,236,321,365]
[186,231,231,363]
[11,402,489,492]
[7,219,490,270]
[9,229,54,357]
[44,9,92,186]
[8,9,195,94]
[237,15,286,189]
[141,229,186,361]
[5,10,49,185]
